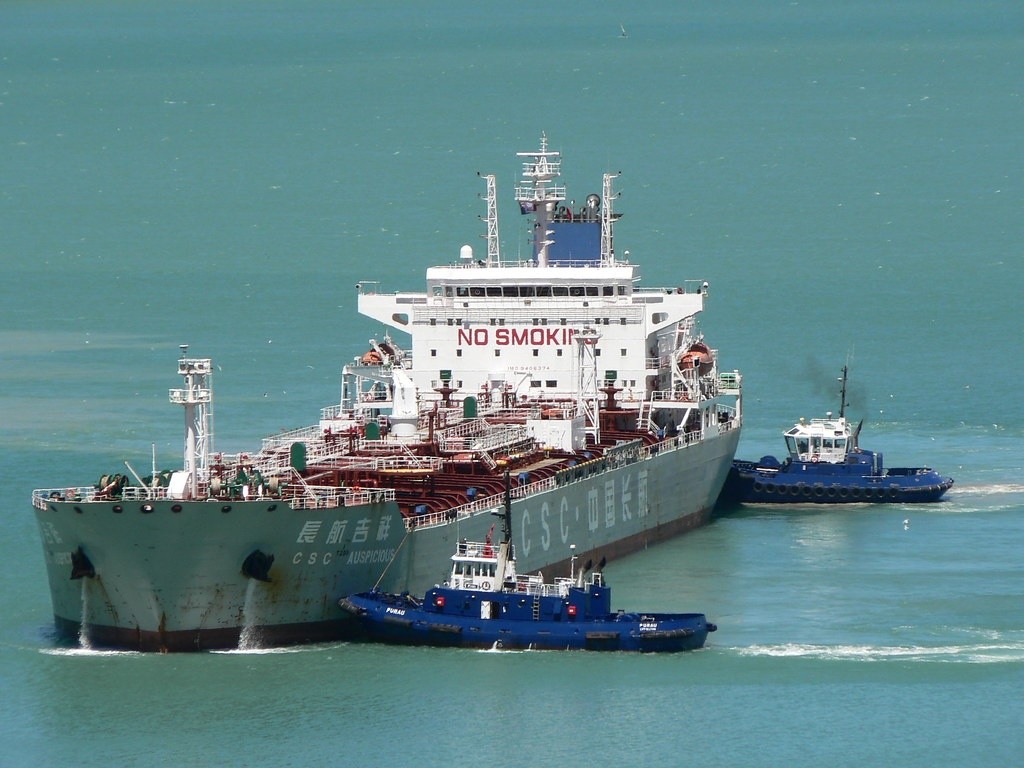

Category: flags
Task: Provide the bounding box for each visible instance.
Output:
[519,202,537,215]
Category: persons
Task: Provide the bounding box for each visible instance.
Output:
[461,538,466,553]
[667,414,674,434]
[799,441,806,454]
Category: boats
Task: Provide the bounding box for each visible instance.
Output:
[720,365,955,503]
[32,130,742,654]
[337,469,718,654]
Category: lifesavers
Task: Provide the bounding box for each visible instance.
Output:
[826,486,839,498]
[752,481,764,493]
[352,486,362,494]
[776,482,789,496]
[810,456,819,463]
[801,484,814,498]
[850,486,863,499]
[788,484,801,497]
[813,484,826,498]
[764,481,777,494]
[875,487,888,499]
[888,486,900,499]
[863,487,876,500]
[837,486,850,498]
